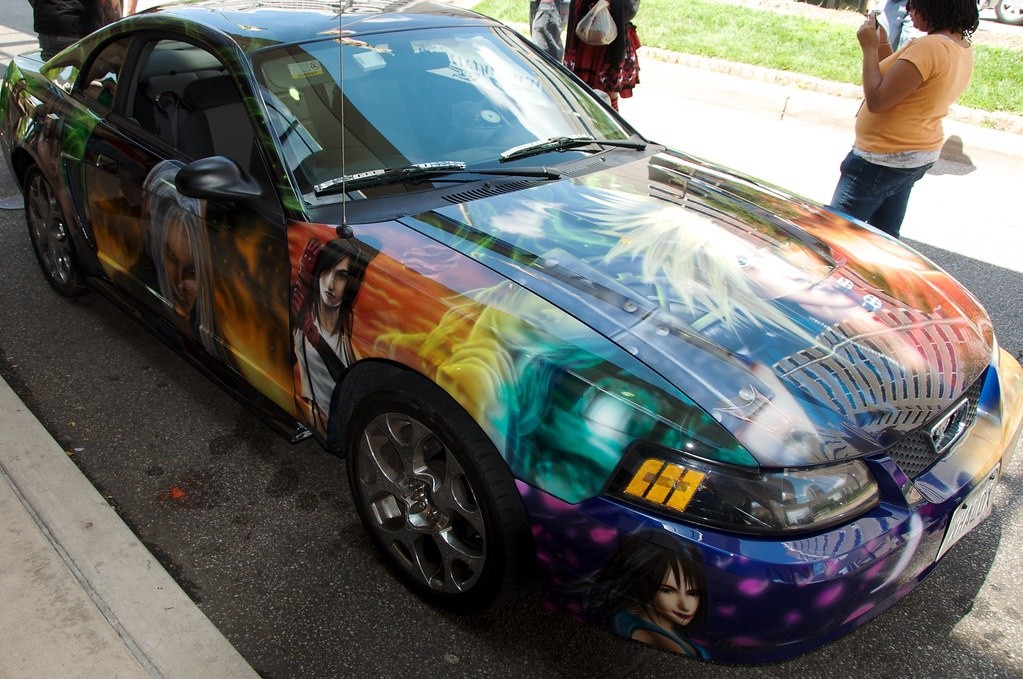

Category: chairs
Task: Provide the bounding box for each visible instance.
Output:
[176,74,264,177]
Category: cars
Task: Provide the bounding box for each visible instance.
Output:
[1,1,1023,666]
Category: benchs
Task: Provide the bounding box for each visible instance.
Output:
[135,41,230,147]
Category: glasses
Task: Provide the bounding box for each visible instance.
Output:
[906,1,913,12]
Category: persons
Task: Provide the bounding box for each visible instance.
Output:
[830,0,979,240]
[28,0,145,62]
[529,0,640,114]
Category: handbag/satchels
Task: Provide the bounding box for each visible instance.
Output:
[575,0,618,46]
